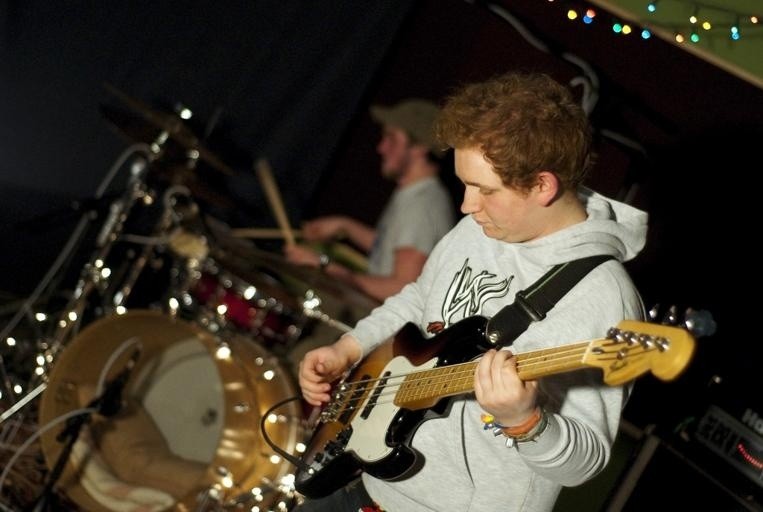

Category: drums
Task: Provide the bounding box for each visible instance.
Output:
[39,310,308,512]
[182,215,308,357]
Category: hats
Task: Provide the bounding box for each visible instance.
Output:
[371,101,440,146]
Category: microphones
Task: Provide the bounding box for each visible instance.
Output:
[187,193,218,248]
[97,350,142,418]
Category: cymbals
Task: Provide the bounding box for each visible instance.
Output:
[93,80,242,215]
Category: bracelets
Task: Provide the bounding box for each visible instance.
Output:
[495,403,548,444]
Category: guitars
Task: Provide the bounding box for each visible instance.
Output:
[294,305,717,501]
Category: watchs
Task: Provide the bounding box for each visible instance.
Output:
[319,253,330,275]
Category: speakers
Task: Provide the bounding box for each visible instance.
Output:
[599,423,763,512]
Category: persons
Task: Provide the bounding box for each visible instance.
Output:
[292,71,648,512]
[283,101,455,304]
